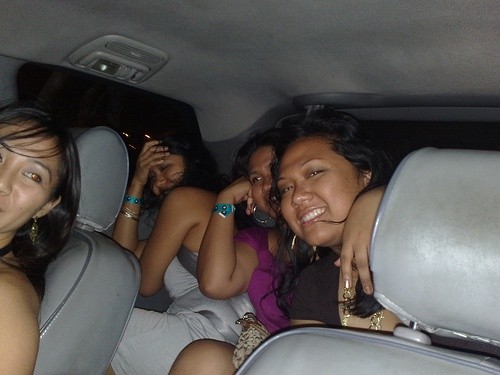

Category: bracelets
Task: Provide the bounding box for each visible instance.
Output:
[212,203,236,218]
[124,194,142,205]
[120,205,139,222]
[216,198,234,201]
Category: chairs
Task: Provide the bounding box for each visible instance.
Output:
[234,147,500,375]
[32,126,141,375]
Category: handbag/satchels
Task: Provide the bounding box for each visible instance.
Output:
[231,312,271,373]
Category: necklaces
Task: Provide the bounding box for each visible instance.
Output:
[342,286,383,330]
[253,214,271,223]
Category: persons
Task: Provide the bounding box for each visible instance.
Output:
[0,102,80,375]
[169,127,319,375]
[269,106,404,333]
[106,132,256,375]
